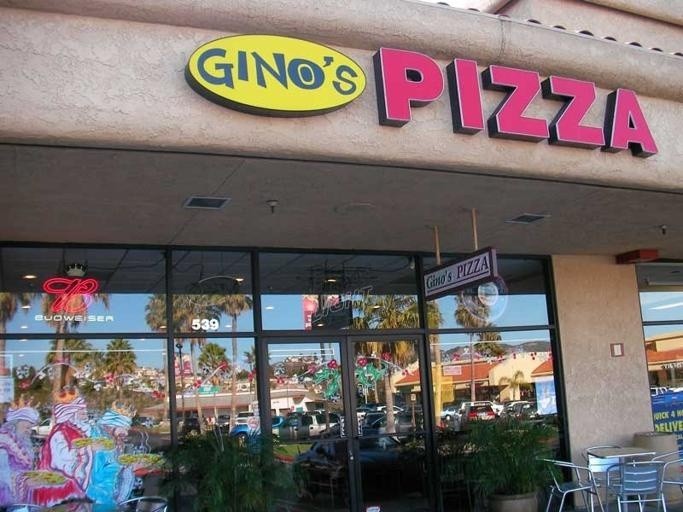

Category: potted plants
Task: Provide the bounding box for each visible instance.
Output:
[436,406,564,512]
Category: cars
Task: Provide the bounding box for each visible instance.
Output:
[133,417,153,428]
[175,417,200,437]
[31,419,51,440]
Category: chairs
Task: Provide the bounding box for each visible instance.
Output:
[536,445,683,511]
